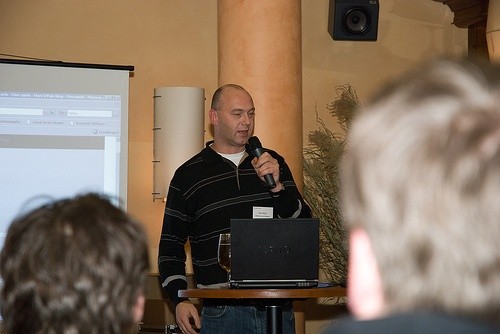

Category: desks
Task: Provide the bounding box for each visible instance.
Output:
[177,288,346,334]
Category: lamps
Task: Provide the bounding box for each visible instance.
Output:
[152,86,206,202]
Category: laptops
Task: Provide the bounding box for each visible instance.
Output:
[220,218,320,289]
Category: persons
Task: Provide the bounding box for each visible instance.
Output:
[157,84,312,334]
[0,192,151,333]
[314,52,499,334]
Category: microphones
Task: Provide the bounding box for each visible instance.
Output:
[248,136,276,189]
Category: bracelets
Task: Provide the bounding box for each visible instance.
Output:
[269,183,285,198]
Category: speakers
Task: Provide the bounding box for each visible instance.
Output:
[328,0,379,41]
